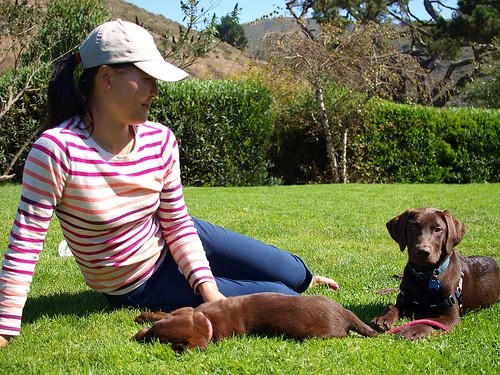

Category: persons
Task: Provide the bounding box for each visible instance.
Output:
[0,16,341,363]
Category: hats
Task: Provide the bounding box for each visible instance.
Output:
[78,19,189,82]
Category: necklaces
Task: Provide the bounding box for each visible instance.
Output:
[82,112,132,158]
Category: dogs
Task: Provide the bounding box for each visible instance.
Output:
[129,292,378,355]
[369,207,500,341]
